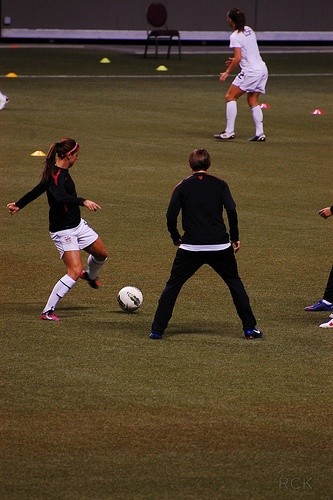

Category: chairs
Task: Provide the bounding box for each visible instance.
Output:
[143,2,182,60]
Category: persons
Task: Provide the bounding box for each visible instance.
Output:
[0,92,9,110]
[148,148,262,340]
[305,206,333,329]
[214,7,268,142]
[6,138,108,321]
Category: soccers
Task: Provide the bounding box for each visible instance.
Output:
[117,286,144,312]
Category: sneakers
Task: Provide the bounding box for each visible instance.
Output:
[79,270,101,289]
[40,310,62,321]
[304,300,333,311]
[148,331,160,340]
[245,327,263,339]
[213,130,236,139]
[249,133,266,141]
[319,318,333,327]
